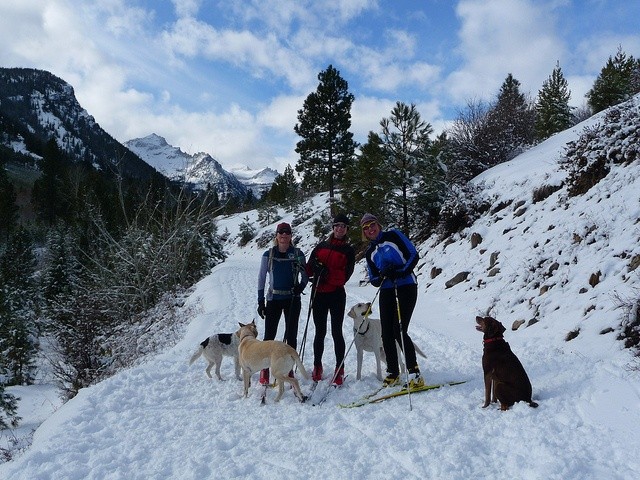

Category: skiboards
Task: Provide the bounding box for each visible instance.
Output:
[301,371,351,406]
[261,378,296,403]
[338,372,467,408]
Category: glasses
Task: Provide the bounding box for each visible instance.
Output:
[362,220,376,230]
[277,229,291,235]
[333,223,349,228]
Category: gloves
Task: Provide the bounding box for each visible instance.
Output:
[291,281,306,296]
[378,273,388,282]
[314,262,329,277]
[311,258,319,272]
[389,267,408,280]
[258,303,267,319]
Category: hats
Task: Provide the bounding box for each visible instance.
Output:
[361,213,378,229]
[277,223,291,231]
[332,213,350,231]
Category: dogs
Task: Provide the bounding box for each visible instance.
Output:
[476,316,540,411]
[238,318,315,403]
[348,302,429,381]
[191,328,244,381]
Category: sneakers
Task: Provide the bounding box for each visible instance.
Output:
[382,372,400,388]
[260,368,269,384]
[312,365,323,381]
[288,369,294,378]
[401,373,425,392]
[333,367,344,384]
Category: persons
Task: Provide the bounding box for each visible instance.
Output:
[306,213,355,386]
[361,213,424,390]
[258,222,309,385]
